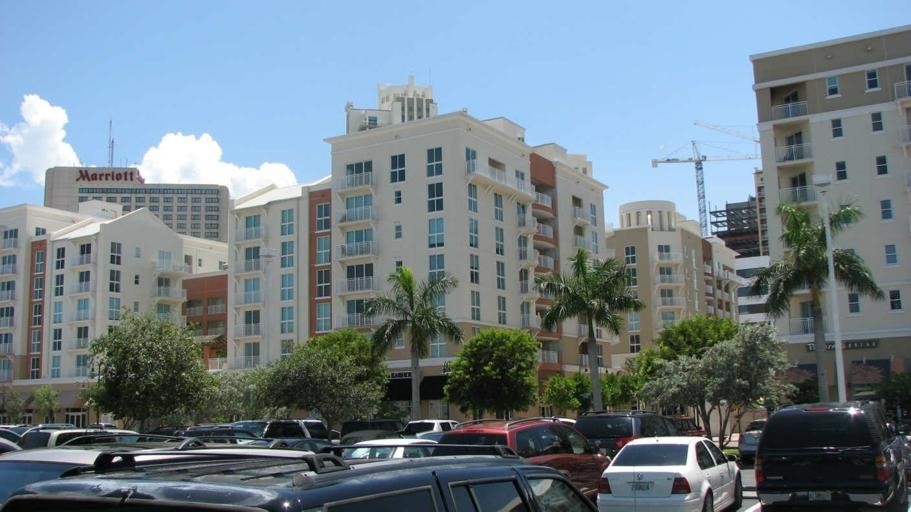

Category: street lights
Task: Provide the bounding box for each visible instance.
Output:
[812,174,848,403]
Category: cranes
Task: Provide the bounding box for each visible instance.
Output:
[651,139,762,236]
[692,120,760,144]
